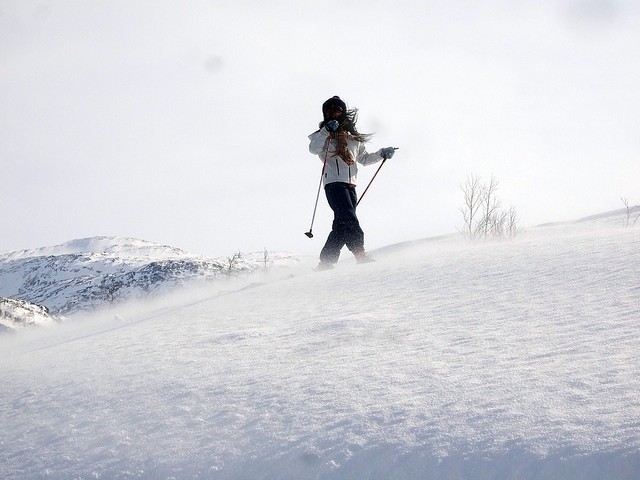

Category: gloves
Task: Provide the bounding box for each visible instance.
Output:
[325,120,340,132]
[381,146,395,159]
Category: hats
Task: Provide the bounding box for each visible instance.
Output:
[323,96,346,115]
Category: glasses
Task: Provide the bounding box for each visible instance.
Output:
[325,105,344,113]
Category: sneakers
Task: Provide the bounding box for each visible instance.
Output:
[314,261,336,271]
[356,251,377,265]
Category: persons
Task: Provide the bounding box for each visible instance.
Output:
[308,96,395,271]
